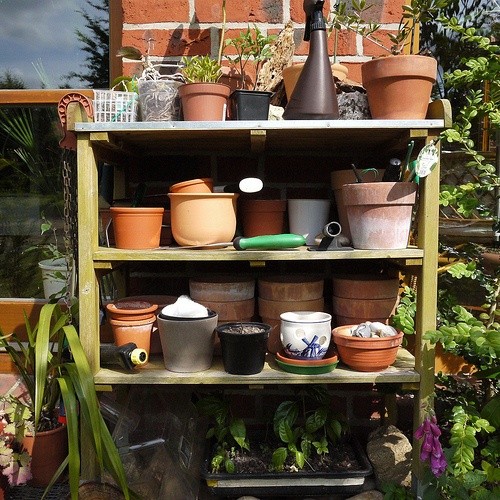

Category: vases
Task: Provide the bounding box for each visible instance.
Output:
[100,161,423,376]
[94,90,139,122]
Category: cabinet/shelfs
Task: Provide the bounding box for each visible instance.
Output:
[76,96,454,500]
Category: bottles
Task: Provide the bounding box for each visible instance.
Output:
[100,341,148,373]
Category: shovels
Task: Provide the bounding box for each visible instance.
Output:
[179,234,305,250]
[308,222,353,250]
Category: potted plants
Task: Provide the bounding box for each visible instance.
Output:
[322,0,450,120]
[0,297,128,500]
[113,45,185,121]
[174,54,235,120]
[227,26,293,120]
[31,218,73,300]
[202,405,373,500]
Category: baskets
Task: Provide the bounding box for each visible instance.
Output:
[92,90,139,123]
